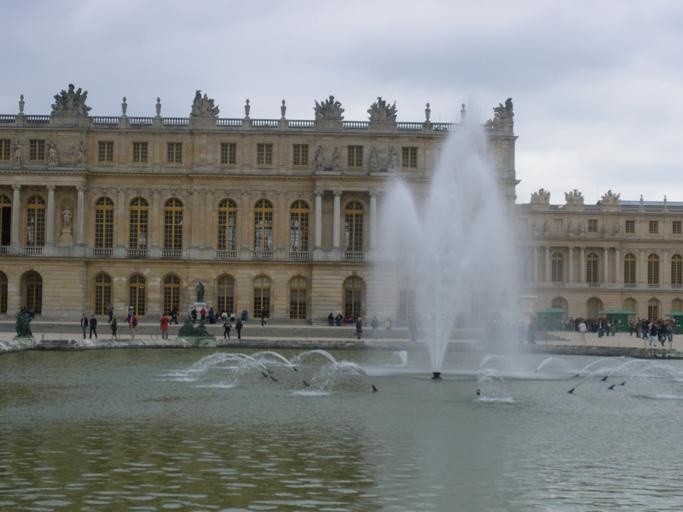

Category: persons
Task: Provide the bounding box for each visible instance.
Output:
[80,310,138,340]
[159,308,249,340]
[327,312,343,326]
[311,144,401,174]
[628,317,674,347]
[194,281,206,303]
[371,316,379,338]
[407,317,419,343]
[260,310,268,327]
[355,317,363,339]
[572,316,617,342]
[13,138,90,231]
